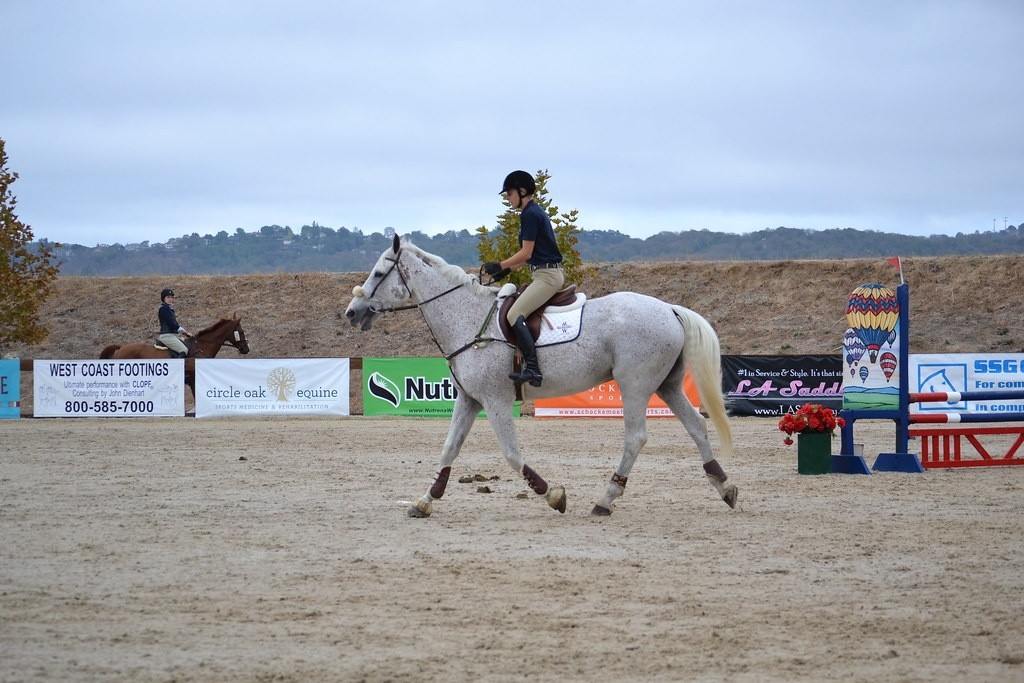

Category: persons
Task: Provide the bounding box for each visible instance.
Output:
[484,169,563,388]
[159,288,193,378]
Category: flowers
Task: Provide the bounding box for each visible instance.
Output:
[778,403,846,446]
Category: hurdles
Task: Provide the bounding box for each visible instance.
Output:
[830,283,1024,474]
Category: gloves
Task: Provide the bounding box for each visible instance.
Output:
[484,262,501,275]
[492,267,512,281]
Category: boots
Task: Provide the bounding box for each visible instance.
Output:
[177,351,187,358]
[508,314,543,387]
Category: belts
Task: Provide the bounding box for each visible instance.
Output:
[529,263,561,271]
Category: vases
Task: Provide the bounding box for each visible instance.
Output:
[797,433,830,475]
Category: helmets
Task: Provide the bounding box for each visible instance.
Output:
[160,289,175,302]
[498,170,536,198]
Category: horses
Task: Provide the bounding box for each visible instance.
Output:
[340,232,743,521]
[97,309,254,402]
